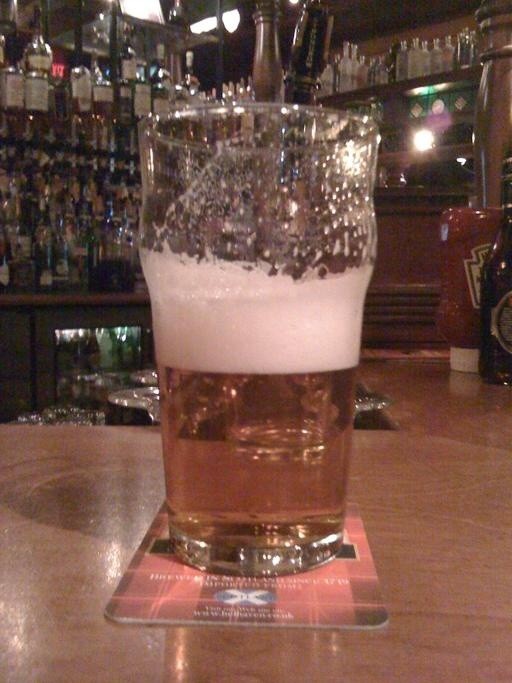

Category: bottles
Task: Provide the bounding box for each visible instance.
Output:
[314,26,478,97]
[437,148,511,386]
[0,0,255,309]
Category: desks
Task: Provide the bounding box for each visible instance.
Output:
[0,357,510,683]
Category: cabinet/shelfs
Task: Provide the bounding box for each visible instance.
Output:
[1,291,154,425]
[314,65,481,199]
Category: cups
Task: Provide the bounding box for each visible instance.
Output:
[136,102,381,579]
